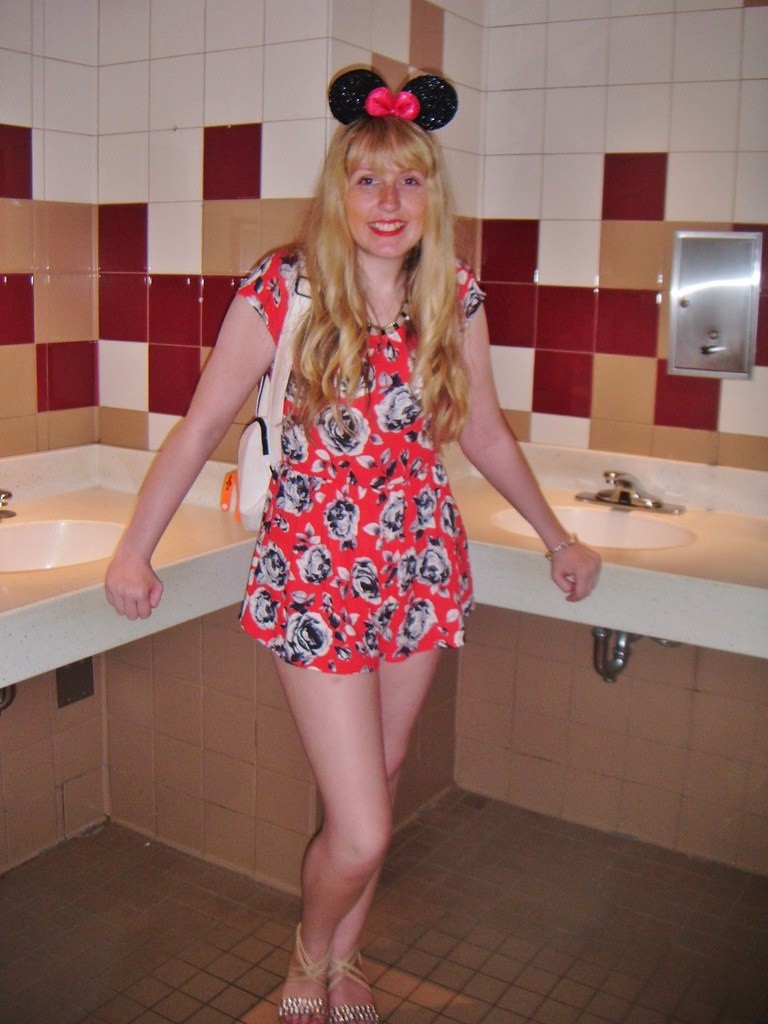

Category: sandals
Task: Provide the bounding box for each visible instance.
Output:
[326,943,379,1024]
[278,922,329,1024]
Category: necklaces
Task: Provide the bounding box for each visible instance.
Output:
[367,300,406,330]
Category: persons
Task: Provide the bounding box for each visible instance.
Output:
[104,69,601,1024]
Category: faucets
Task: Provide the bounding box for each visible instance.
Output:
[0,488,16,518]
[594,470,663,509]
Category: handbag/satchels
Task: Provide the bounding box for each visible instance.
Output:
[220,248,321,531]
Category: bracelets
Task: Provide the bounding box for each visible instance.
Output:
[544,533,578,561]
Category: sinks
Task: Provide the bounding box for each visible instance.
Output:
[489,504,699,550]
[0,520,128,574]
